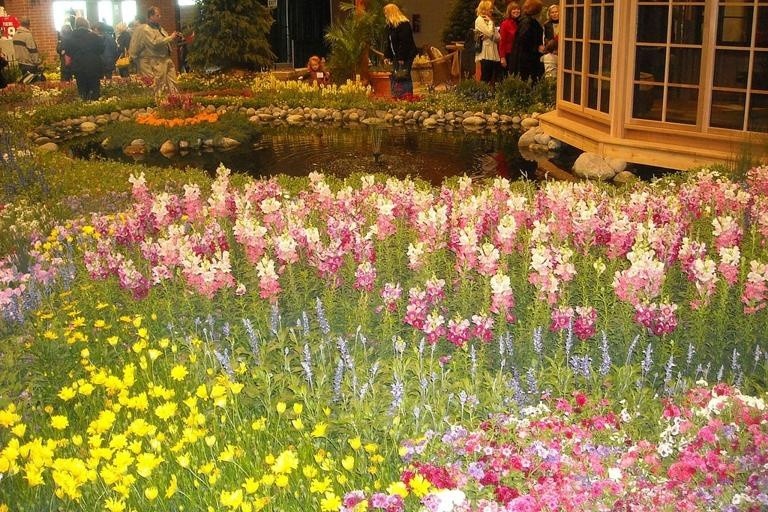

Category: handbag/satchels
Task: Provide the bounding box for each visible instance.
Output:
[19,71,46,85]
[115,47,130,70]
[392,64,409,81]
[64,55,71,68]
[464,29,482,53]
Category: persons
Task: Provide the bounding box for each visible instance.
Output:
[474,0,559,90]
[13,16,47,84]
[382,4,419,99]
[297,54,331,89]
[54,5,185,107]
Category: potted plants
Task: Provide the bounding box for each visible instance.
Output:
[340,2,394,97]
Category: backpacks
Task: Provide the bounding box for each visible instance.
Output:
[128,24,155,59]
